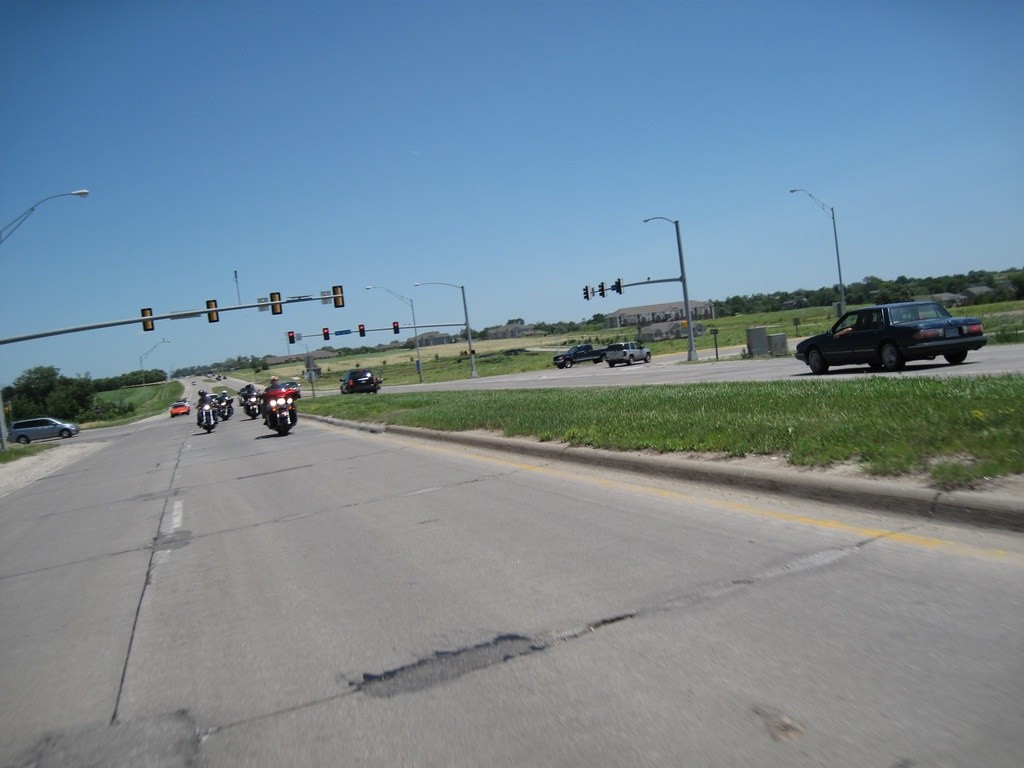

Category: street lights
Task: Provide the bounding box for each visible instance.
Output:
[366,282,479,384]
[139,340,171,417]
[790,189,846,329]
[642,216,698,362]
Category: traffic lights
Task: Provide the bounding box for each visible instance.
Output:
[393,322,399,334]
[615,278,623,294]
[288,328,330,344]
[359,325,365,337]
[599,282,606,297]
[583,285,591,301]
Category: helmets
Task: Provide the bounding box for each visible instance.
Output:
[248,384,254,388]
[222,390,228,394]
[198,390,205,396]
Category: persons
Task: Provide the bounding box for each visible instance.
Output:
[196,390,218,425]
[216,390,234,415]
[371,374,379,394]
[262,376,297,429]
[833,313,876,339]
[242,383,262,416]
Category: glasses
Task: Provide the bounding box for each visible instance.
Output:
[273,379,279,382]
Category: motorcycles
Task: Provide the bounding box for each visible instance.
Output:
[196,395,299,436]
[241,391,261,419]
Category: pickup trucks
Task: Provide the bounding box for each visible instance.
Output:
[553,344,607,369]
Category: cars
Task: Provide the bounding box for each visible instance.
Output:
[280,382,301,398]
[237,388,258,405]
[605,342,651,367]
[6,398,190,444]
[339,368,377,394]
[794,301,988,374]
